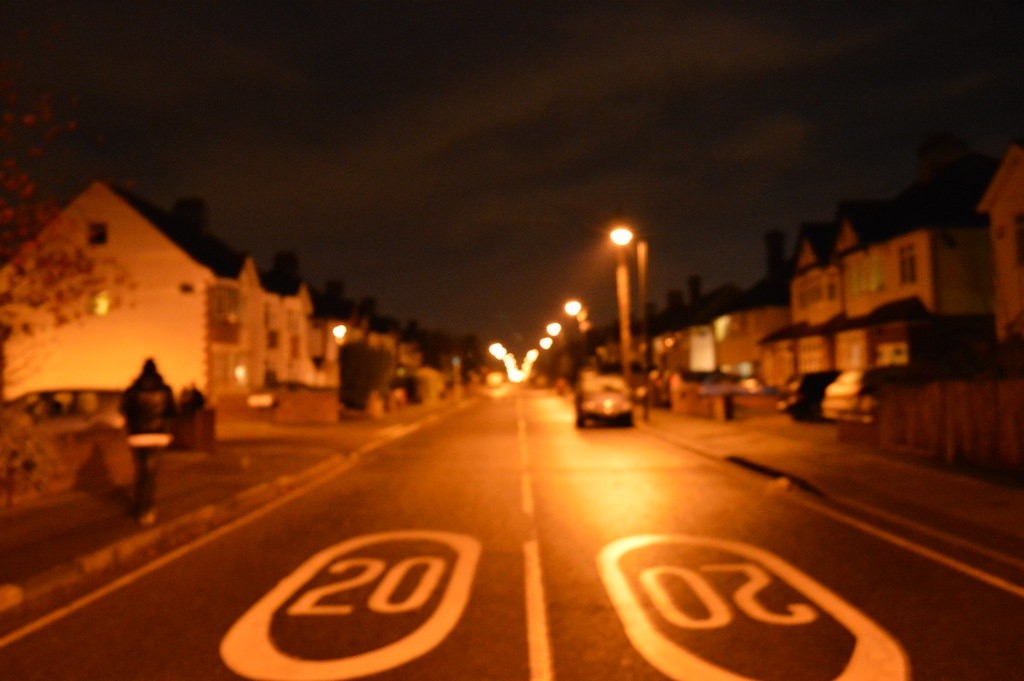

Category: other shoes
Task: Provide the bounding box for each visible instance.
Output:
[138,511,157,526]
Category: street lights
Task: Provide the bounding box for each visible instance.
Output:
[610,227,651,378]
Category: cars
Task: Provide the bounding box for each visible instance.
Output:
[575,372,634,428]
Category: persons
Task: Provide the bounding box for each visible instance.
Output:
[118,358,175,525]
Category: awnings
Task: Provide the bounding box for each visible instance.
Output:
[758,294,919,343]
